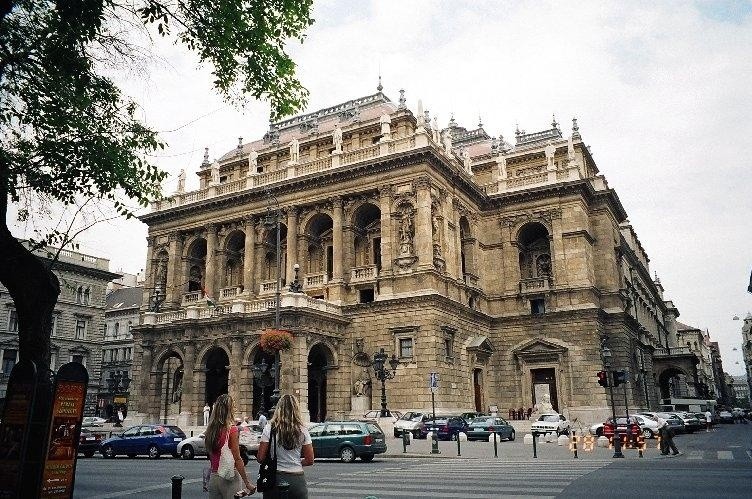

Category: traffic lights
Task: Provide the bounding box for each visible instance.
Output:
[597,370,610,387]
[613,370,624,386]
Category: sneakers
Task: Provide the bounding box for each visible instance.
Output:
[665,451,683,458]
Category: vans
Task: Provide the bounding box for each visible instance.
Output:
[300,420,387,463]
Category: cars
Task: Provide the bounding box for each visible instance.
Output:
[531,413,571,436]
[590,407,752,446]
[78,428,102,459]
[99,423,185,460]
[81,418,105,427]
[361,409,516,443]
[176,421,264,458]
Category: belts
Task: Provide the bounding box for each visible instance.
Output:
[276,469,305,477]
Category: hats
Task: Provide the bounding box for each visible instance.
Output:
[257,411,263,414]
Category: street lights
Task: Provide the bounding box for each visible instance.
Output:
[105,370,131,423]
[263,192,284,413]
[250,359,276,419]
[600,345,624,458]
[372,348,399,417]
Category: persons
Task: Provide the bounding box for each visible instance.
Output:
[704,408,716,433]
[651,414,680,455]
[203,393,257,499]
[200,401,211,427]
[256,394,316,499]
[257,410,268,435]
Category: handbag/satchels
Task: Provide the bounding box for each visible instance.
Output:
[216,423,240,481]
[202,459,212,492]
[667,427,676,438]
[256,420,277,492]
